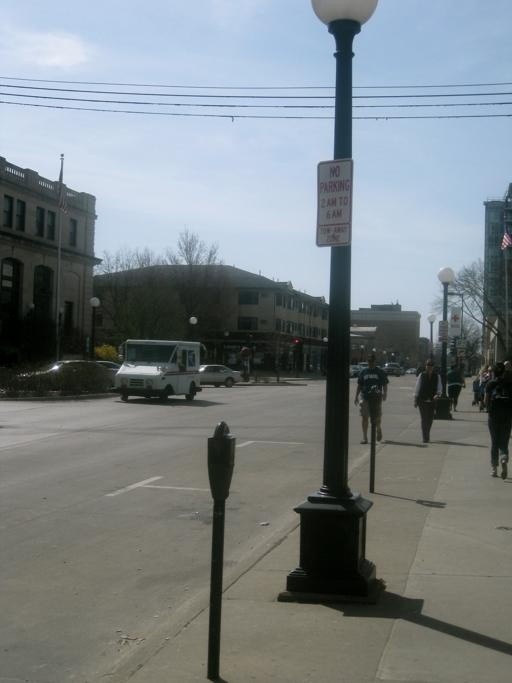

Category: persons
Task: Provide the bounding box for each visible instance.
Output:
[483,360,510,479]
[354,353,389,443]
[415,359,511,412]
[414,358,443,443]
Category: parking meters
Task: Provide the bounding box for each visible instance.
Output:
[206,419,236,679]
[367,381,380,493]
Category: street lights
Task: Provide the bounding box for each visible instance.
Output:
[188,315,198,340]
[359,344,410,366]
[89,297,101,359]
[323,335,328,377]
[282,0,390,612]
[426,313,436,366]
[431,265,455,417]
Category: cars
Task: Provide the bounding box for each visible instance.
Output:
[350,361,417,377]
[13,336,243,403]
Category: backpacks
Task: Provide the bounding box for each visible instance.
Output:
[491,383,512,423]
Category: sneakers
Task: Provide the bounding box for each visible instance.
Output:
[377,428,382,441]
[360,438,368,444]
[490,468,497,477]
[501,459,507,480]
[423,432,429,443]
